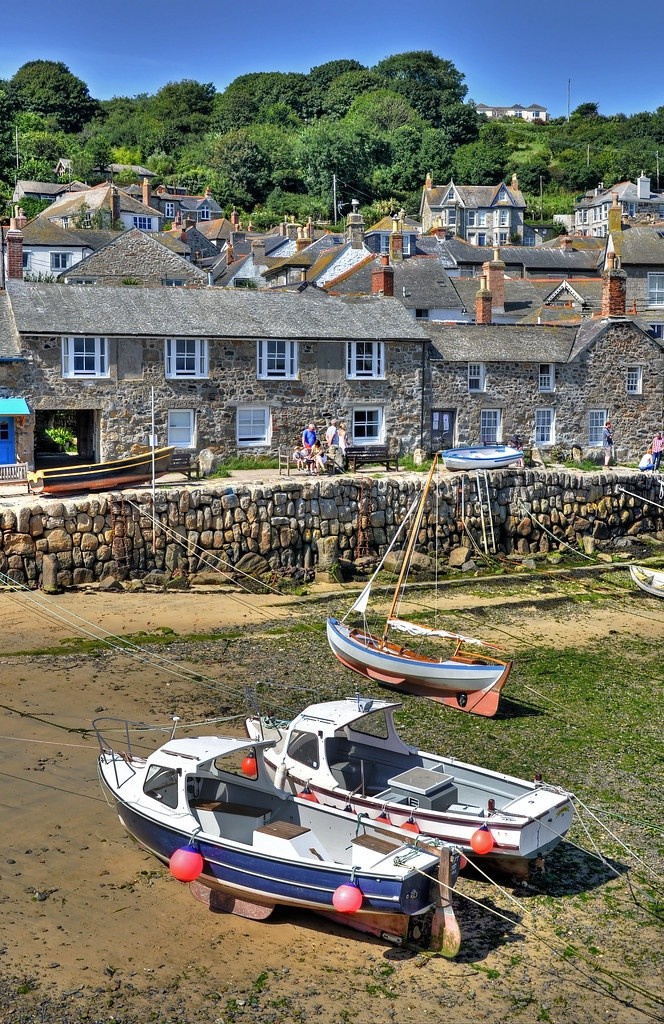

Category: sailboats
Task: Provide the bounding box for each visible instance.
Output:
[326,450,514,717]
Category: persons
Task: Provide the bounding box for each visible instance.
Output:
[602,422,615,467]
[293,446,305,471]
[507,434,525,467]
[302,424,317,473]
[307,439,327,477]
[638,450,655,473]
[326,419,339,446]
[338,421,350,456]
[651,432,664,473]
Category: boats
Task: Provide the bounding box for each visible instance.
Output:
[630,565,664,598]
[245,679,576,861]
[443,444,524,472]
[26,444,177,497]
[90,712,466,959]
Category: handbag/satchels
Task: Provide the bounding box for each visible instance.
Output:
[306,459,314,463]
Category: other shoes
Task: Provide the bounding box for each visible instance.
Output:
[322,468,328,473]
[309,472,317,476]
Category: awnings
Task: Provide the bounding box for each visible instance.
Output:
[0,399,33,416]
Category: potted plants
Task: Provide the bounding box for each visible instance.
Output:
[42,427,75,452]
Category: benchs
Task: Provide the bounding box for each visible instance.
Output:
[190,799,273,846]
[149,453,201,484]
[350,835,400,865]
[485,442,533,467]
[344,446,399,473]
[278,447,336,476]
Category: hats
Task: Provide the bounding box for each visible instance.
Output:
[309,424,315,427]
[331,419,340,423]
[315,440,321,445]
[294,446,300,450]
